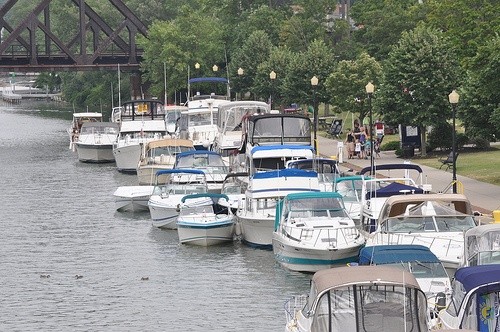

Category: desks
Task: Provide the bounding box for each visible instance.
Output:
[318,118,328,129]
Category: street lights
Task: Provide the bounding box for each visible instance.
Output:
[194,63,201,95]
[213,65,219,94]
[448,90,460,192]
[365,80,375,174]
[237,67,244,101]
[270,70,276,110]
[311,75,318,154]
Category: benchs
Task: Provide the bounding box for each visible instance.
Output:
[438,151,459,171]
[318,123,331,125]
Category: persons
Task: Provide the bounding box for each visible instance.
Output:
[307,106,313,114]
[344,122,380,160]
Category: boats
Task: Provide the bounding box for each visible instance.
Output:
[177,193,236,247]
[357,194,500,264]
[217,99,339,183]
[236,145,320,248]
[146,169,209,229]
[463,223,500,267]
[283,264,428,332]
[114,186,152,214]
[69,62,231,185]
[288,157,426,235]
[426,262,500,332]
[357,244,452,312]
[270,191,366,274]
